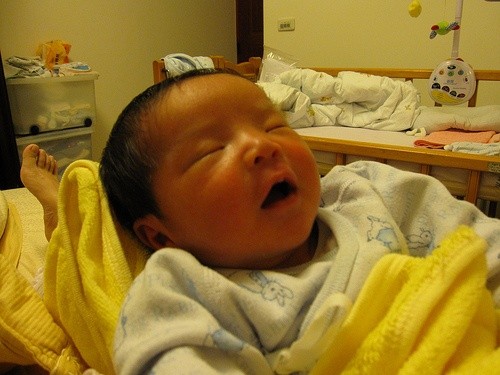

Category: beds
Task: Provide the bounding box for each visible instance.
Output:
[152,56,500,218]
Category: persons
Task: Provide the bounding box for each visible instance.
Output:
[20,144,60,243]
[99,67,500,375]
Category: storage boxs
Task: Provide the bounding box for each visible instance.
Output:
[6,74,98,135]
[17,127,94,178]
